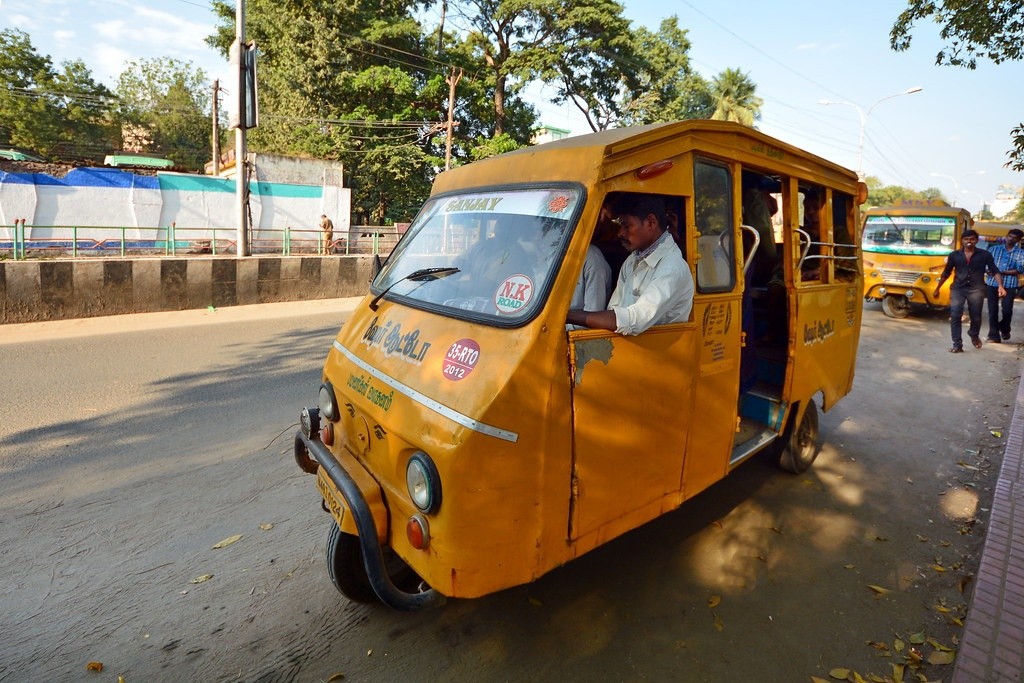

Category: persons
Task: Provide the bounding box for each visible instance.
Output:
[442,194,694,338]
[319,214,334,255]
[759,187,860,344]
[932,229,1007,354]
[985,228,1024,344]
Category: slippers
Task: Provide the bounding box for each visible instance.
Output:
[968,329,982,348]
[948,346,962,353]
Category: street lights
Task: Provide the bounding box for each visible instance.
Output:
[817,86,923,182]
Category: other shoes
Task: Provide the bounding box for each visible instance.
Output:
[1003,330,1010,340]
[985,338,1001,344]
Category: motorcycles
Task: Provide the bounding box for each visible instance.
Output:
[292,118,868,613]
[859,206,975,319]
[972,221,1024,300]
